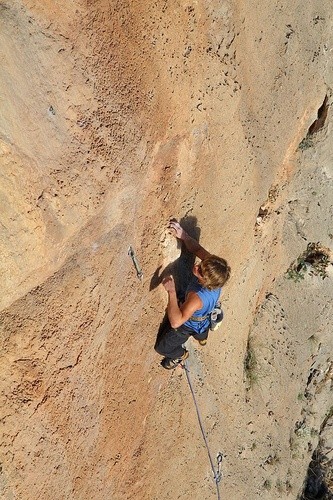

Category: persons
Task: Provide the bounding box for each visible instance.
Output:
[154,220,232,370]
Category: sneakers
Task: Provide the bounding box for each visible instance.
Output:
[161,349,189,370]
[199,338,207,346]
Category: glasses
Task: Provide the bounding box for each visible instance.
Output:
[198,259,204,277]
